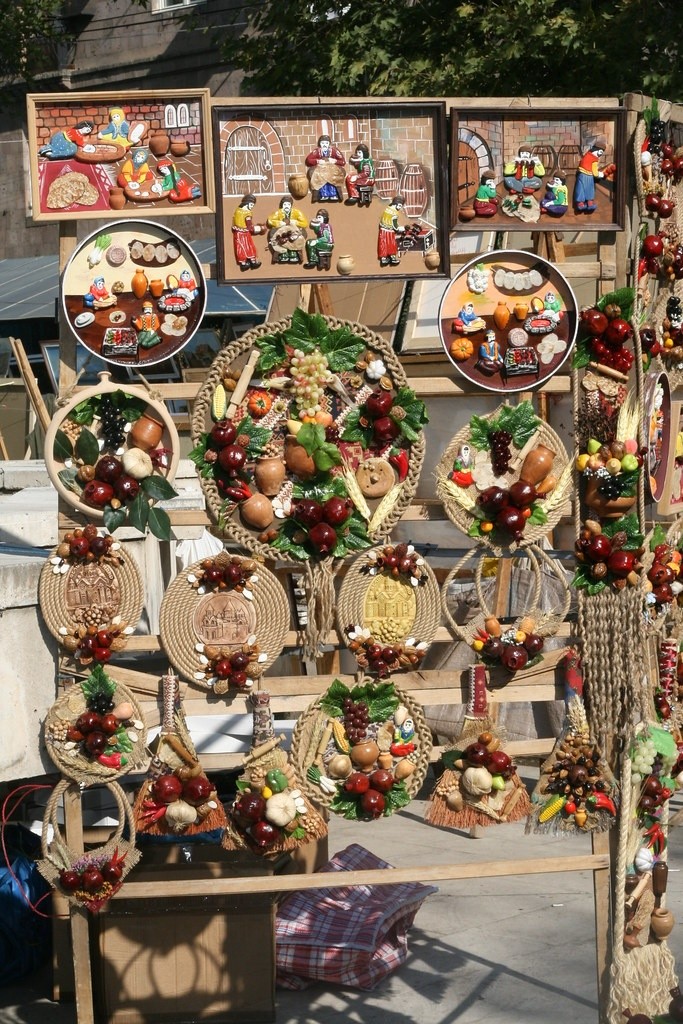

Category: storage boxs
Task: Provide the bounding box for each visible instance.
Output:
[98,860,277,1024]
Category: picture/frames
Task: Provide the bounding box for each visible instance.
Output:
[26,86,215,222]
[212,99,449,287]
[448,104,629,235]
